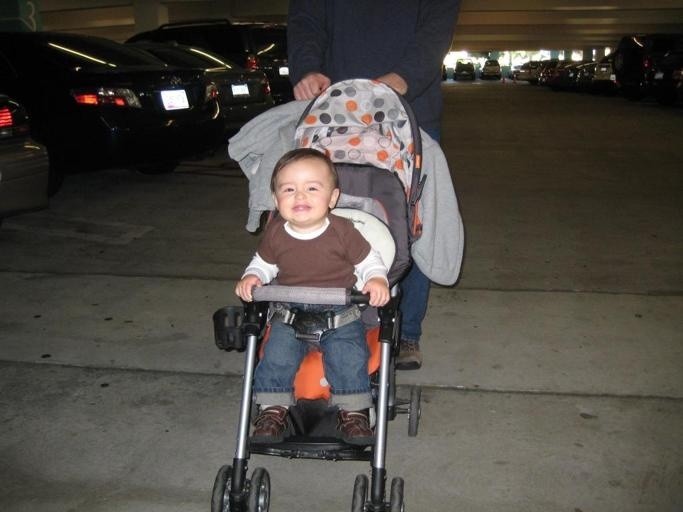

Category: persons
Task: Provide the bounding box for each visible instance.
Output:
[233,147,392,447]
[284,1,459,371]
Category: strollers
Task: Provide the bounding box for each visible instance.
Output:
[204,73,428,510]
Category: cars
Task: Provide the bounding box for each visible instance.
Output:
[0,94,51,216]
[0,30,221,200]
[507,49,620,87]
[643,43,682,108]
[118,38,277,137]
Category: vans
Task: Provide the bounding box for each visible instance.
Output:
[482,59,500,80]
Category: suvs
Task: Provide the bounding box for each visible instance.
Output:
[120,17,289,101]
[453,59,475,80]
[611,32,682,100]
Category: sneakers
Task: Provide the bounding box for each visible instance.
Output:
[395,339,422,370]
[250,406,295,446]
[336,408,375,447]
